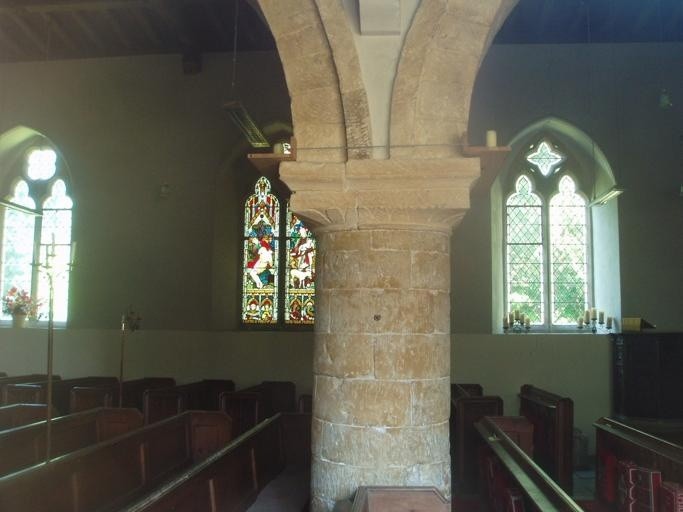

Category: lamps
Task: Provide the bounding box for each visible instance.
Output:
[582,1,623,211]
[219,0,272,149]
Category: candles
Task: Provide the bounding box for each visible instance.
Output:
[273,143,282,154]
[486,130,497,148]
[502,309,530,329]
[578,308,613,327]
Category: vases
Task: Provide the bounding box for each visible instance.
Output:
[12,314,27,328]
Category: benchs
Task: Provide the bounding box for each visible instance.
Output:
[0,371,295,512]
[450,382,683,511]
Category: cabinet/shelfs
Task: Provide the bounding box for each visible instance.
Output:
[610,330,683,419]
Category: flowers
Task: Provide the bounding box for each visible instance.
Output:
[0,286,43,321]
